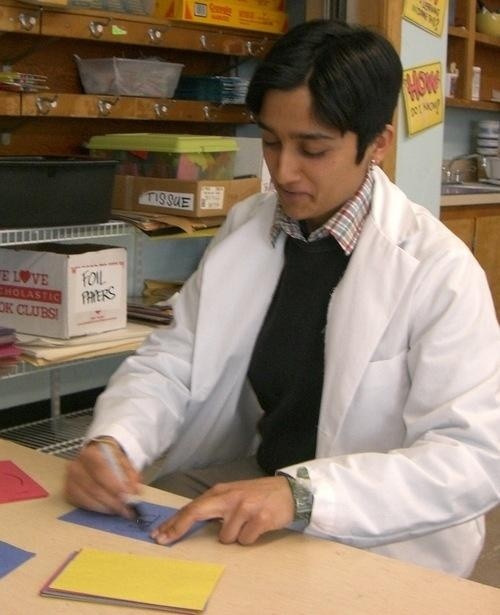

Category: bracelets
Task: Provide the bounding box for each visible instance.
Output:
[274,468,320,537]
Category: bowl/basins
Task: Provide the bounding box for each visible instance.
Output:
[477,14,500,35]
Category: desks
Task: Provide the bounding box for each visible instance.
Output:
[0,435,500,615]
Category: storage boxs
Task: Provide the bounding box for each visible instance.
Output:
[110,173,261,218]
[0,153,122,229]
[85,132,239,181]
[0,243,129,341]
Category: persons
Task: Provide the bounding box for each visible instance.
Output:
[55,16,500,579]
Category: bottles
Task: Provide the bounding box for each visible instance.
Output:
[472,64,480,101]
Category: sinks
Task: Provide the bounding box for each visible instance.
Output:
[441,185,500,196]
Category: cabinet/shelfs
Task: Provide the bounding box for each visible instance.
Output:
[445,0,500,112]
[0,0,332,412]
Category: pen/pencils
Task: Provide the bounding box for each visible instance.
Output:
[0,71,48,92]
[101,441,153,533]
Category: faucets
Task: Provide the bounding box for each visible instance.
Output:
[449,154,486,170]
